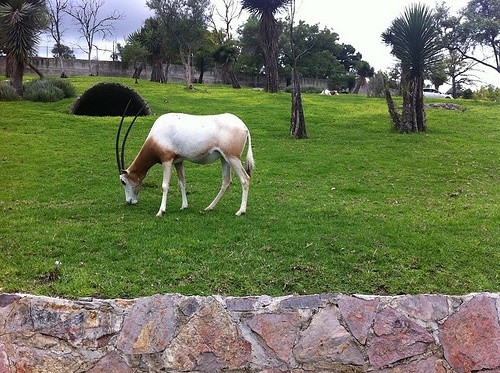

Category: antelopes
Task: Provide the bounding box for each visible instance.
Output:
[320,88,339,96]
[115,100,255,218]
